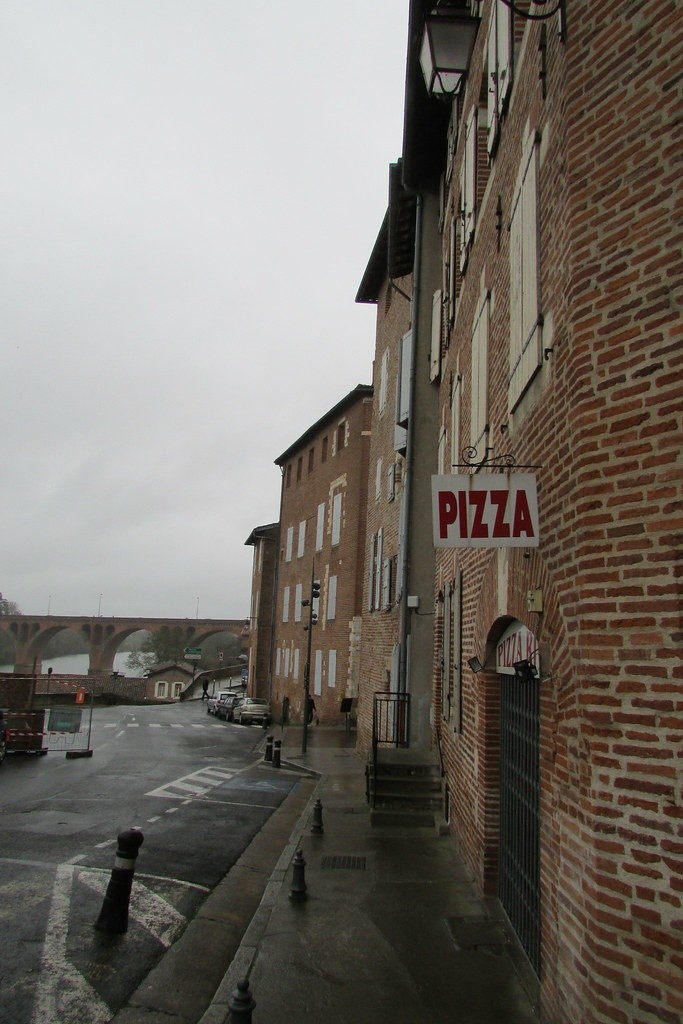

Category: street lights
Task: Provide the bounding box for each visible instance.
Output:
[301,554,320,754]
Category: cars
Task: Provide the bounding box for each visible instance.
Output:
[206,691,272,725]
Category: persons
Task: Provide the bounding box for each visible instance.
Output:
[282,696,288,722]
[307,694,317,727]
[201,677,210,701]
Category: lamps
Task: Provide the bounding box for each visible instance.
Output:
[512,649,540,683]
[415,0,567,104]
[467,650,493,674]
